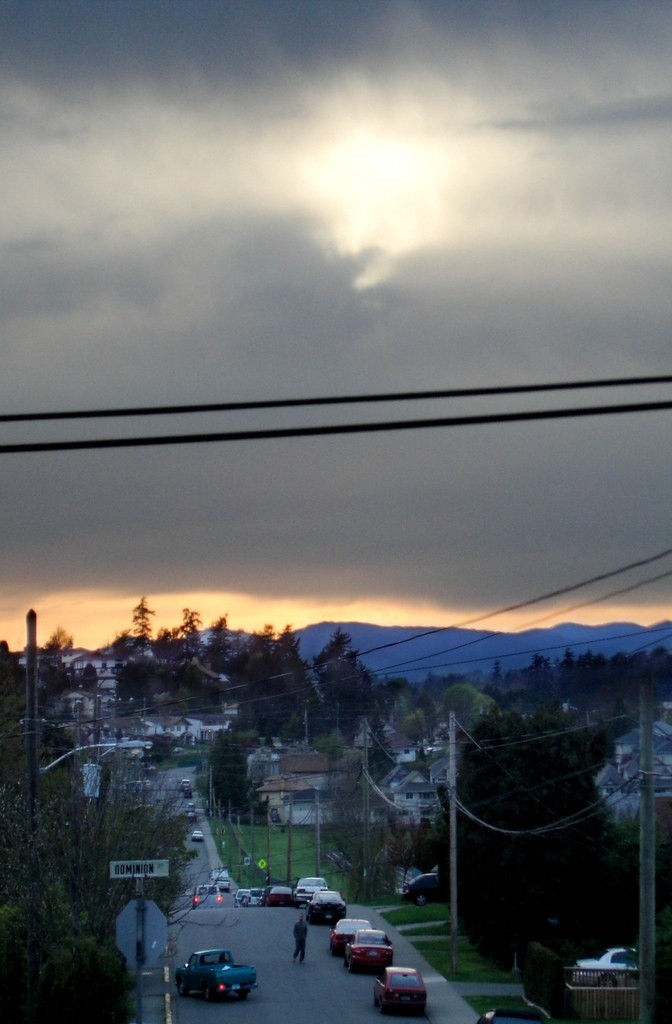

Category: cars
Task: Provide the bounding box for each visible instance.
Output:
[329,916,373,956]
[193,868,295,909]
[295,877,329,906]
[401,871,442,907]
[180,779,197,823]
[477,1008,547,1024]
[192,831,203,841]
[305,889,347,924]
[344,929,394,974]
[372,965,426,1017]
[571,947,640,988]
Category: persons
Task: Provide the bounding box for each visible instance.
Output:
[293,913,307,964]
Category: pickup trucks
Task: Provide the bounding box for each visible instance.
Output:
[174,948,259,1002]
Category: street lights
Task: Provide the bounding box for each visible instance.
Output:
[24,741,146,1021]
[91,741,154,815]
